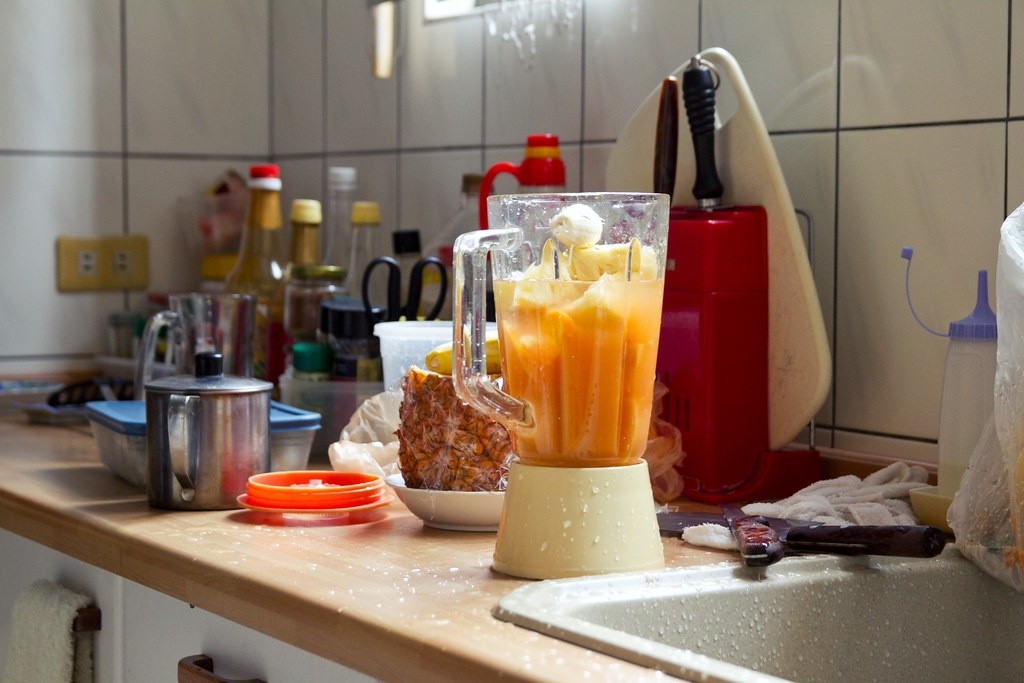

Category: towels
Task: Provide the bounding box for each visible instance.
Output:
[4,578,94,683]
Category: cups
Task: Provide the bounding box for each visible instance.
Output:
[145,352,274,511]
[132,290,255,398]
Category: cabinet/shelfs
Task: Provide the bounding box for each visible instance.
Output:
[0,531,386,683]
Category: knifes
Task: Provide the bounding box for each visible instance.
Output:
[722,505,784,569]
[655,512,943,559]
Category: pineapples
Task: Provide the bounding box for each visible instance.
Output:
[398,366,514,491]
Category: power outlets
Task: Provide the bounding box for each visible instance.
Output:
[57,235,151,292]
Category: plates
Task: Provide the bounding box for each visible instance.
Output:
[0,379,87,423]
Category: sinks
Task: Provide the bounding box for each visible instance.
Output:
[491,540,1023,682]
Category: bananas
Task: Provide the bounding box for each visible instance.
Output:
[549,204,602,248]
[427,333,502,375]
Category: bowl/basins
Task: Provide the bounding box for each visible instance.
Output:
[909,486,954,532]
[374,321,500,395]
[85,398,321,492]
[385,474,506,532]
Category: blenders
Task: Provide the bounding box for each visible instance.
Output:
[450,190,671,579]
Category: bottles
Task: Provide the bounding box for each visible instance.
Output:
[225,163,490,413]
[900,246,999,495]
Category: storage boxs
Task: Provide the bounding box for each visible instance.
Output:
[85,396,323,492]
[280,377,385,459]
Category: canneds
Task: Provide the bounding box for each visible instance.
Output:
[285,266,347,337]
